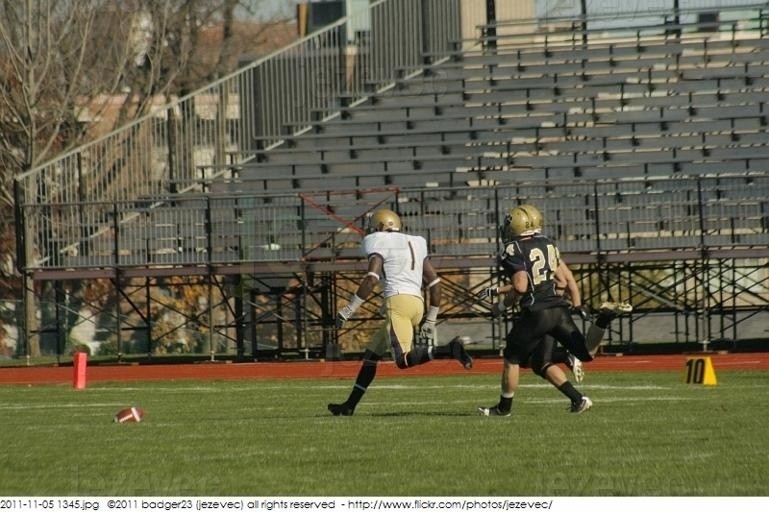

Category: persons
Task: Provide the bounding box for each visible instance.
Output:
[327,209,474,416]
[482,224,584,382]
[477,203,633,421]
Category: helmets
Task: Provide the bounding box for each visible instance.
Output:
[507,204,545,239]
[366,210,402,234]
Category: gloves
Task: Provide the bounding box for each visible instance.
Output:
[420,321,436,340]
[478,288,499,301]
[335,304,355,330]
[575,304,590,322]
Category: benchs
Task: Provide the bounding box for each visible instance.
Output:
[57,0,769,268]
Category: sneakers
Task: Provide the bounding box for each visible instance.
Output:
[449,337,473,370]
[563,351,585,384]
[476,406,512,418]
[565,396,593,415]
[600,300,632,318]
[327,403,354,417]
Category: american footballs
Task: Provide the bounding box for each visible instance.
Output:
[112,406,143,424]
